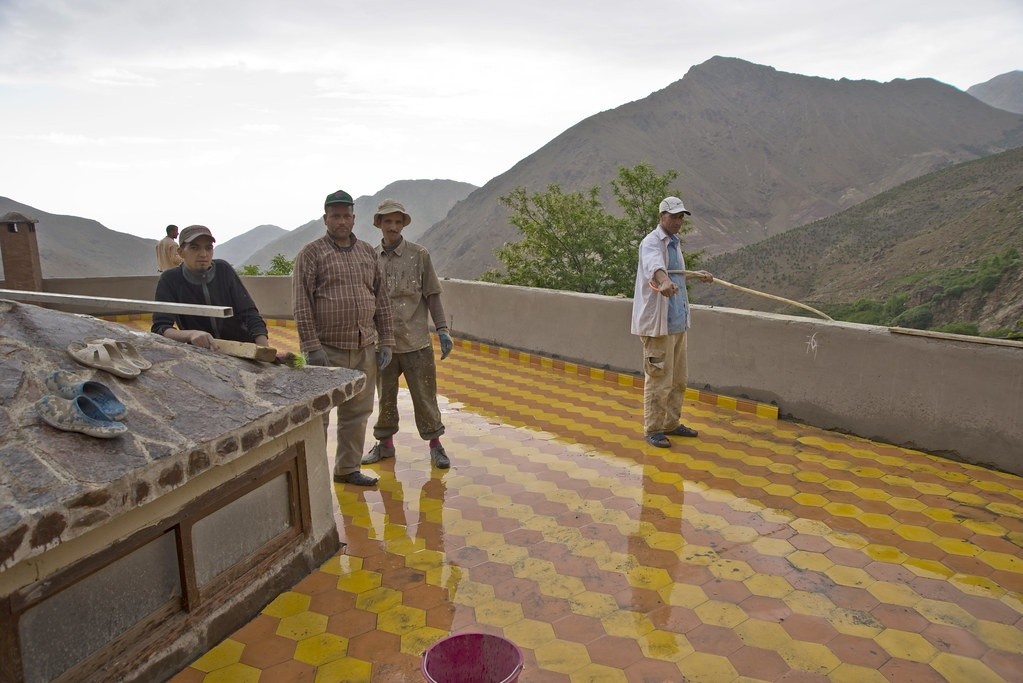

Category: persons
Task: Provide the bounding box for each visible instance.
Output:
[156,225,184,277]
[150,225,287,365]
[631,196,714,447]
[292,190,396,486]
[362,199,453,470]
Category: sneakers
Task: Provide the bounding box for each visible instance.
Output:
[430,444,450,468]
[664,424,698,437]
[361,442,395,463]
[645,433,671,448]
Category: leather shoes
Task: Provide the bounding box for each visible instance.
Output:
[334,471,378,486]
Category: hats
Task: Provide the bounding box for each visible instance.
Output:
[659,197,692,216]
[179,224,215,247]
[325,190,355,207]
[374,199,411,229]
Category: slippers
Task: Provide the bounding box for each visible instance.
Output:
[68,342,141,379]
[45,370,128,421]
[35,395,128,438]
[82,336,153,371]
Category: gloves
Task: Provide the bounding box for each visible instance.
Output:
[438,330,453,360]
[308,349,330,367]
[378,346,392,369]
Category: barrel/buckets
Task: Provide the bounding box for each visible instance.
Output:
[418,630,525,683]
